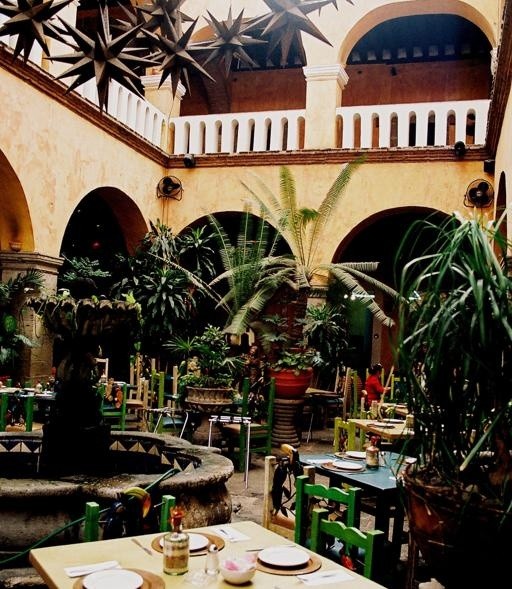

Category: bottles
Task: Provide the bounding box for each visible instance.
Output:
[204,544,220,575]
[101,372,107,383]
[365,436,379,470]
[35,380,42,394]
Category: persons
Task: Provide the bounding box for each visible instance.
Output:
[366,365,386,403]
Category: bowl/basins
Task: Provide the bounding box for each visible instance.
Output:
[219,564,255,586]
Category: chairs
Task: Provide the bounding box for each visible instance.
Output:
[332,356,407,454]
[261,443,316,542]
[83,494,177,542]
[291,474,362,562]
[309,506,388,581]
[1,356,163,432]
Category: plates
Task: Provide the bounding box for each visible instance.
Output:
[82,569,144,589]
[257,546,311,568]
[345,450,365,459]
[159,533,210,551]
[373,422,392,426]
[383,419,401,422]
[333,461,362,470]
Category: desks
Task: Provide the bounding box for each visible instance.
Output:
[297,449,431,560]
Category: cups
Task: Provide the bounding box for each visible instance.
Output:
[162,533,189,575]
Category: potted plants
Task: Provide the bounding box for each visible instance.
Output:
[261,305,345,398]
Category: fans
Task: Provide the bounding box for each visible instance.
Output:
[466,178,495,209]
[156,175,185,202]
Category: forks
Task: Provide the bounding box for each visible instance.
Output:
[220,529,234,540]
[293,573,335,582]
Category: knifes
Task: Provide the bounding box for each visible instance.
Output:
[132,538,153,556]
[246,545,296,552]
